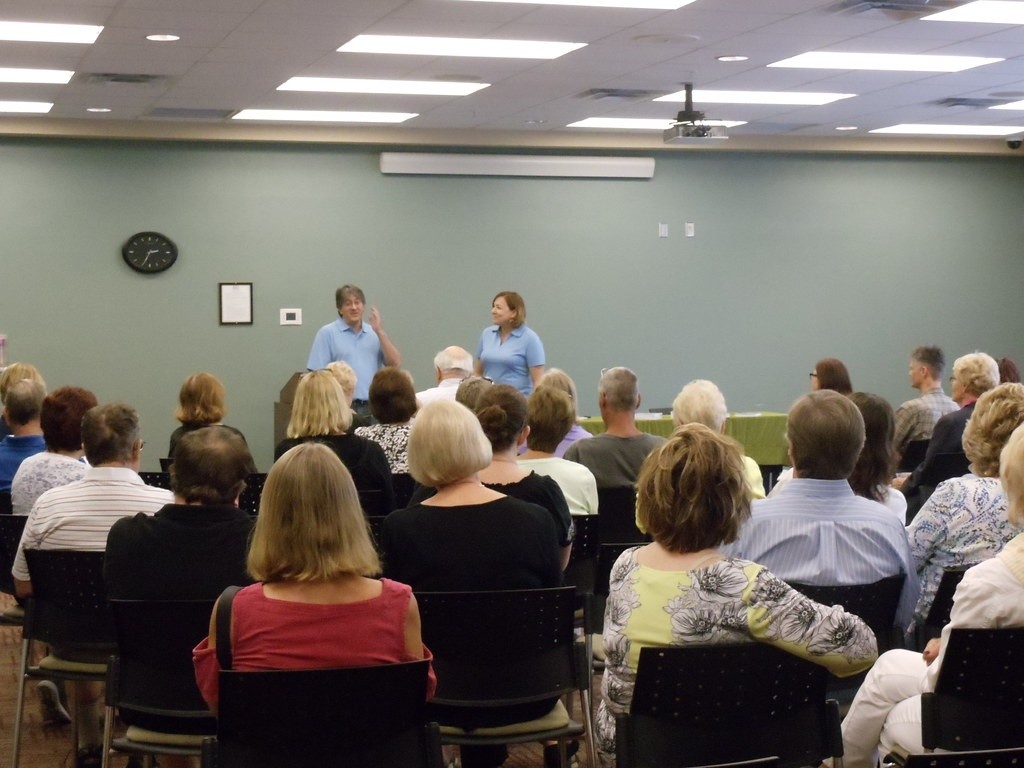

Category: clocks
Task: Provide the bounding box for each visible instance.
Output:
[122,231,178,274]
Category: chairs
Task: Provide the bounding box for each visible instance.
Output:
[0,431,1024,768]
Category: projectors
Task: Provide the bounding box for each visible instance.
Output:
[664,124,729,144]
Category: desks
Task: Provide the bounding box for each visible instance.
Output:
[567,407,796,487]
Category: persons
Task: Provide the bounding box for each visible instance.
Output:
[474,291,545,398]
[0,345,1024,768]
[819,421,1024,768]
[562,366,671,489]
[904,381,1024,653]
[594,421,879,768]
[10,385,98,516]
[381,397,580,768]
[306,284,401,426]
[167,373,248,458]
[104,424,257,737]
[716,388,923,650]
[192,441,437,719]
[11,401,175,725]
[273,367,397,548]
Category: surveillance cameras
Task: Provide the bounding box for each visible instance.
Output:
[1006,138,1023,149]
